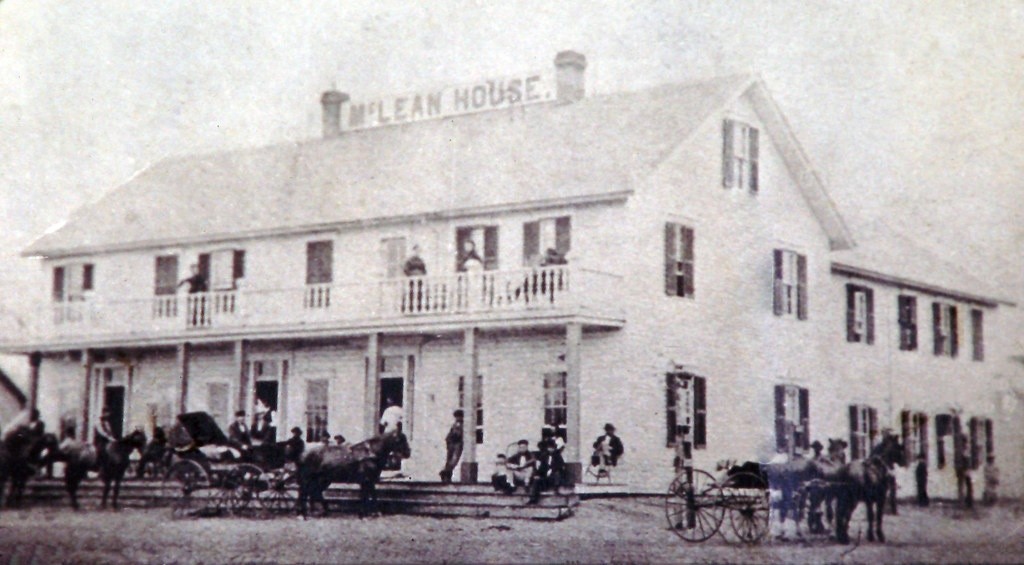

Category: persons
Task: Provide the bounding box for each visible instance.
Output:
[176,263,207,325]
[168,419,191,447]
[252,390,277,441]
[93,406,116,470]
[587,423,624,485]
[457,238,482,273]
[774,438,849,540]
[515,247,567,298]
[278,427,305,462]
[333,435,352,449]
[379,395,403,434]
[915,452,930,506]
[438,410,463,480]
[6,409,43,504]
[491,439,536,496]
[135,426,166,480]
[227,409,252,465]
[954,433,1001,508]
[528,422,565,505]
[402,244,427,311]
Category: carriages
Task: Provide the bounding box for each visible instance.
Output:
[160,411,412,518]
[664,429,910,548]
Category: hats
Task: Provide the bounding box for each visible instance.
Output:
[292,427,302,435]
[810,441,823,449]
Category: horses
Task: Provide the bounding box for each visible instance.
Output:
[727,429,908,546]
[37,427,146,512]
[295,421,412,521]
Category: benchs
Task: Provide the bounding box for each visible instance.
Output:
[193,441,231,464]
